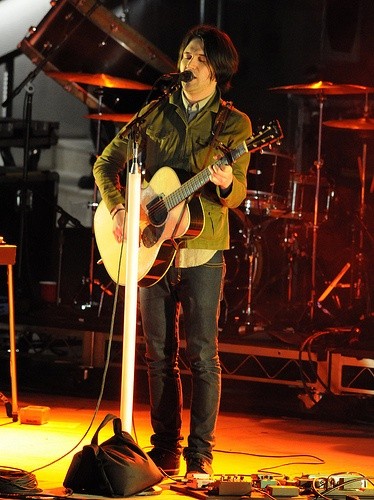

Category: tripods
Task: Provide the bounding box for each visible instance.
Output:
[72,88,116,308]
[253,91,374,340]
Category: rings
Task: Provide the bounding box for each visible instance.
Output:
[120,236,123,239]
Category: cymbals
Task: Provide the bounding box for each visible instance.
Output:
[88,114,139,122]
[266,80,374,97]
[45,70,155,90]
[320,119,374,130]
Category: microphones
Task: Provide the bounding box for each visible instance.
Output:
[160,71,194,82]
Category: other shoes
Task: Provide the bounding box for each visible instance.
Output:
[146,449,180,475]
[184,456,214,482]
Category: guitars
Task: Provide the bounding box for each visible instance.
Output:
[91,116,288,288]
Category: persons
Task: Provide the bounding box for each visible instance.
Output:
[92,24,253,477]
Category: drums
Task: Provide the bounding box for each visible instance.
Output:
[18,0,180,123]
[241,148,295,210]
[265,173,335,220]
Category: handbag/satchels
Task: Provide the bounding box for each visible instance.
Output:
[63,414,164,497]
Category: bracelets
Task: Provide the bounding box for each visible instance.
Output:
[112,208,124,219]
